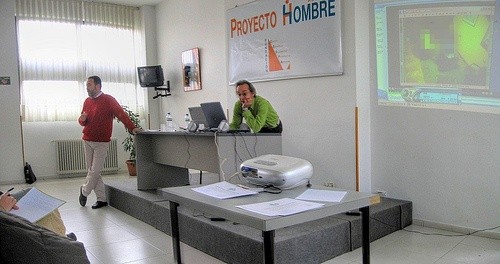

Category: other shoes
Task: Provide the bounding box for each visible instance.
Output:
[79,186,87,206]
[92,200,108,209]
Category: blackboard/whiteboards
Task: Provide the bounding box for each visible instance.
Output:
[224,0,344,86]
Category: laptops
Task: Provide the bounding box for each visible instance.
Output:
[201,102,251,132]
[188,106,209,131]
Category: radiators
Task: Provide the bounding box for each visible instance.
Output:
[50,138,118,178]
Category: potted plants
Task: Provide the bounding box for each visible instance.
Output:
[115,104,143,176]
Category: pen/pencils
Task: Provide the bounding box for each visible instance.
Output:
[1,187,14,201]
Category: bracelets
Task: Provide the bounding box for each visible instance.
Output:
[243,108,248,110]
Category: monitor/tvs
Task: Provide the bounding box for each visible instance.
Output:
[136,64,163,87]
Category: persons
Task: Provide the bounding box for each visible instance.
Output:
[0,193,91,264]
[228,80,283,134]
[78,76,143,209]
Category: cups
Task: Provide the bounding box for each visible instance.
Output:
[160,124,165,132]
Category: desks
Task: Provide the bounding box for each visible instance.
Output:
[161,184,380,264]
[134,130,282,191]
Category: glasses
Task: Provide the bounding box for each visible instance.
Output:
[238,90,251,97]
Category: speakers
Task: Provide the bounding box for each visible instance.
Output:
[218,120,230,133]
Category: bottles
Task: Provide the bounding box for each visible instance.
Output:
[165,113,172,131]
[184,114,190,129]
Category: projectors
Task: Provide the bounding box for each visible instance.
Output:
[241,153,314,189]
